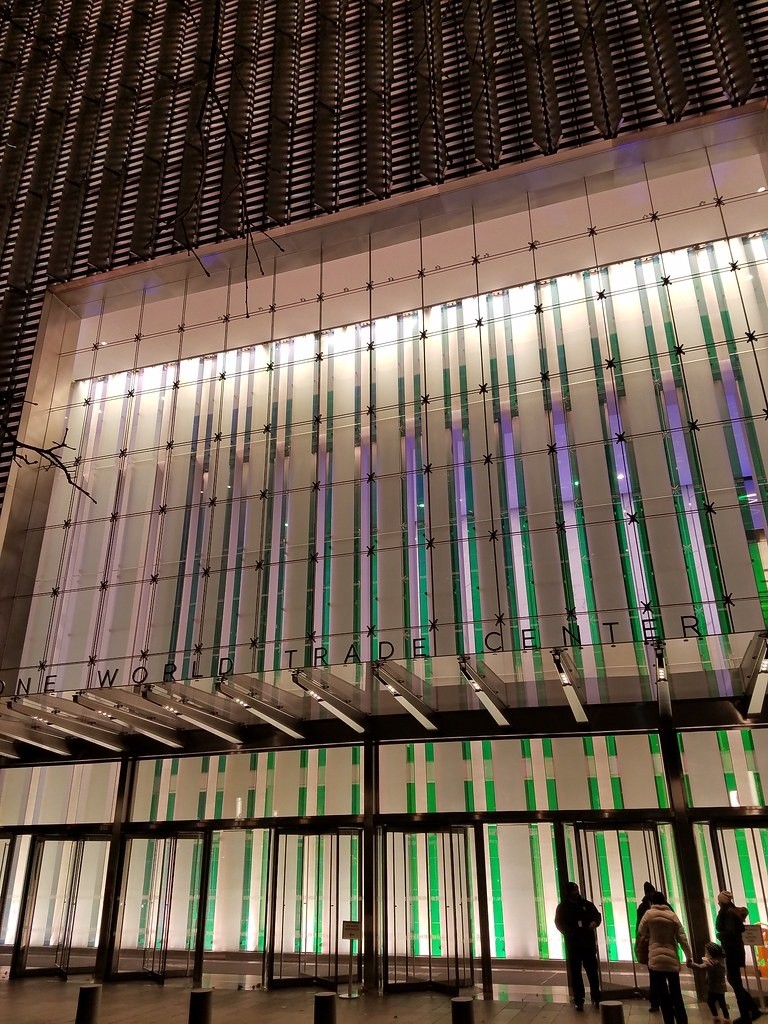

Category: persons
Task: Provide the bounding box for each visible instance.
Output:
[716,891,761,1023]
[640,893,693,1024]
[690,942,731,1024]
[555,883,603,1009]
[636,881,675,1011]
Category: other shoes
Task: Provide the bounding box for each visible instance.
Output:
[648,1006,660,1013]
[723,1018,732,1024]
[591,1001,599,1009]
[574,1004,585,1011]
[752,1011,762,1021]
[732,1016,752,1024]
[713,1018,721,1024]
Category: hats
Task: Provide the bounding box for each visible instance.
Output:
[642,882,655,896]
[717,890,734,904]
